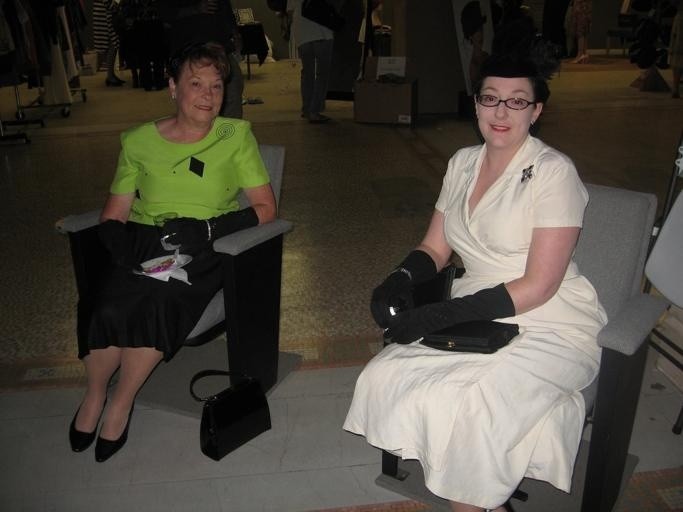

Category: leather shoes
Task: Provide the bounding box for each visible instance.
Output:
[94,398,135,462]
[69,386,107,452]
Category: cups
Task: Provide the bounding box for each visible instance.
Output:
[153,212,182,250]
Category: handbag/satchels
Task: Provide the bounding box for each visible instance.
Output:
[419,321,519,355]
[189,369,271,461]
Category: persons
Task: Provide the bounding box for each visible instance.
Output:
[91,1,594,124]
[66,39,277,463]
[341,48,609,512]
[666,0,683,100]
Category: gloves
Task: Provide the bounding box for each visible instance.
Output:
[102,218,149,272]
[382,283,516,344]
[370,249,437,326]
[160,206,259,255]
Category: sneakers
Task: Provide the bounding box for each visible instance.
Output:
[105,76,126,87]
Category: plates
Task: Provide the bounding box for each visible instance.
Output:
[132,254,193,276]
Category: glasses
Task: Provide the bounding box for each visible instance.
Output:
[476,95,537,110]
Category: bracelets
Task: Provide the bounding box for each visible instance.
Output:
[387,267,413,284]
[203,220,214,241]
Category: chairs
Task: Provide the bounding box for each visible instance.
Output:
[379,183,673,512]
[53,141,296,417]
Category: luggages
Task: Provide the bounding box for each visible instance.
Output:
[139,44,166,92]
